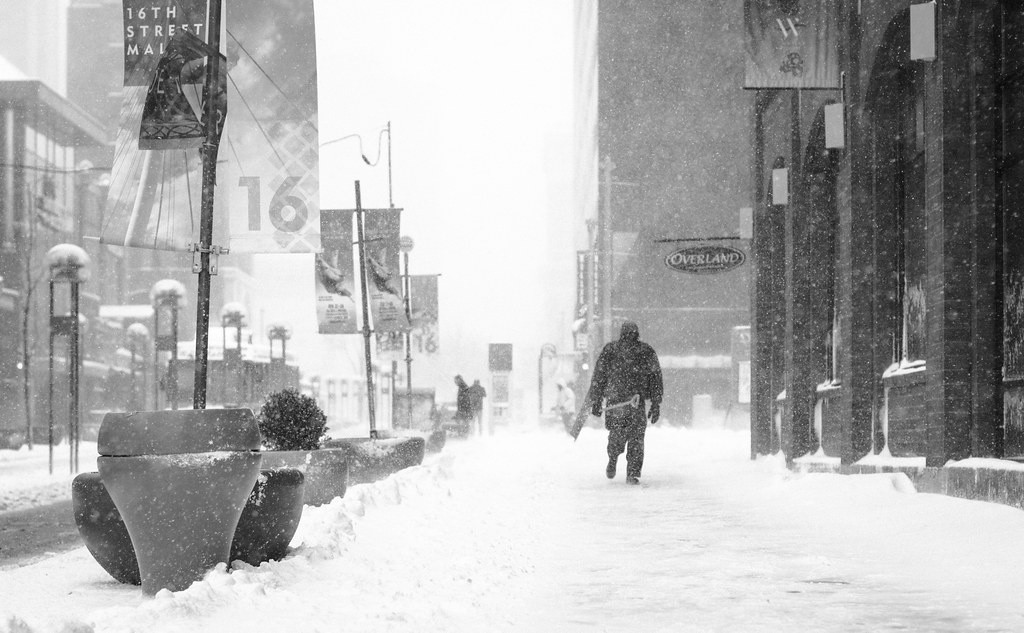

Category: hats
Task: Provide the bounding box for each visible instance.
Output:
[621,320,639,334]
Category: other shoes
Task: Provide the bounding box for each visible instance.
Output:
[606,460,618,479]
[626,476,639,484]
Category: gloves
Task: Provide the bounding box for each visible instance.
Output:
[648,401,661,423]
[592,399,603,417]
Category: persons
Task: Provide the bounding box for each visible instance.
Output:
[550,381,577,436]
[470,379,487,435]
[452,374,473,441]
[588,320,663,486]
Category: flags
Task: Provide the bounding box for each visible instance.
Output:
[93,0,440,353]
[742,0,843,90]
